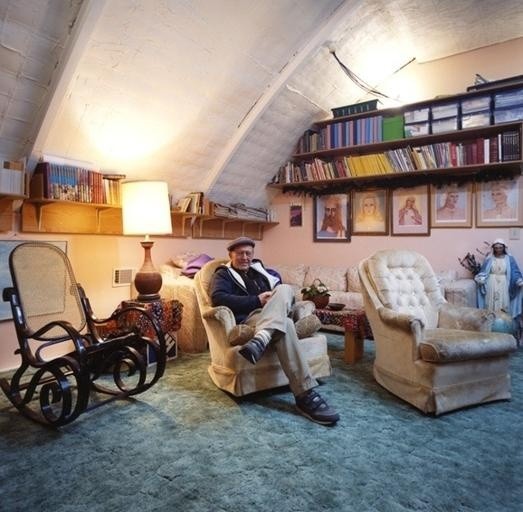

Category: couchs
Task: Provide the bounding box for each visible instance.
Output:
[265,262,477,338]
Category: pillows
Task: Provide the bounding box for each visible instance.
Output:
[170,248,214,268]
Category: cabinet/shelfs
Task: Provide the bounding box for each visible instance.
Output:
[272,77,521,187]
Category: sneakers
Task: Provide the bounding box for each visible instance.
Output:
[238,334,269,366]
[295,389,340,426]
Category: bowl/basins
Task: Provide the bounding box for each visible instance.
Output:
[327,303,345,312]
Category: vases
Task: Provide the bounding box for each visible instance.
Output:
[302,294,329,309]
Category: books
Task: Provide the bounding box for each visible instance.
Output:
[168,189,278,224]
[0,158,122,206]
[264,82,522,186]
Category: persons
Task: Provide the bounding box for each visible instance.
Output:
[475,239,522,321]
[206,236,341,427]
[397,193,423,227]
[315,196,347,238]
[435,187,465,221]
[352,192,385,233]
[481,185,517,220]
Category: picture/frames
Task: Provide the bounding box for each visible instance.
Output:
[429,180,473,228]
[475,175,522,228]
[311,189,351,243]
[390,182,431,236]
[351,187,390,237]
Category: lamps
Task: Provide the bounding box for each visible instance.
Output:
[119,178,173,300]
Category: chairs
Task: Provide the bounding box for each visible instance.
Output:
[0,241,167,430]
[193,257,334,402]
[129,252,208,353]
[357,247,517,417]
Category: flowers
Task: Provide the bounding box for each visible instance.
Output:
[299,279,332,298]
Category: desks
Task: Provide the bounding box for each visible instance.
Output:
[118,300,183,367]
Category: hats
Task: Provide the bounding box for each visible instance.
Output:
[226,237,255,251]
